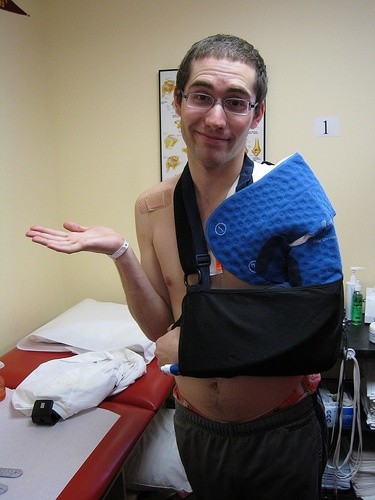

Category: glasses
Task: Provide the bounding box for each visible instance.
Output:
[181,89,259,115]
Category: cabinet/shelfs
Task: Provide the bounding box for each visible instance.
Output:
[324,315,375,500]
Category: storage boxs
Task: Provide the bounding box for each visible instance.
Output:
[318,380,354,427]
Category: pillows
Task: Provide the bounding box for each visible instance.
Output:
[122,409,193,493]
[16,298,157,365]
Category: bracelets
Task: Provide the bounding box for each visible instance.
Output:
[107,237,130,262]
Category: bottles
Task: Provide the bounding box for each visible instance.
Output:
[351,287,362,325]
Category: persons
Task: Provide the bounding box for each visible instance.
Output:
[25,33,345,500]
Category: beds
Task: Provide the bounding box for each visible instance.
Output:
[0,340,175,500]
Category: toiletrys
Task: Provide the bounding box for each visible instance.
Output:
[345,266,366,326]
